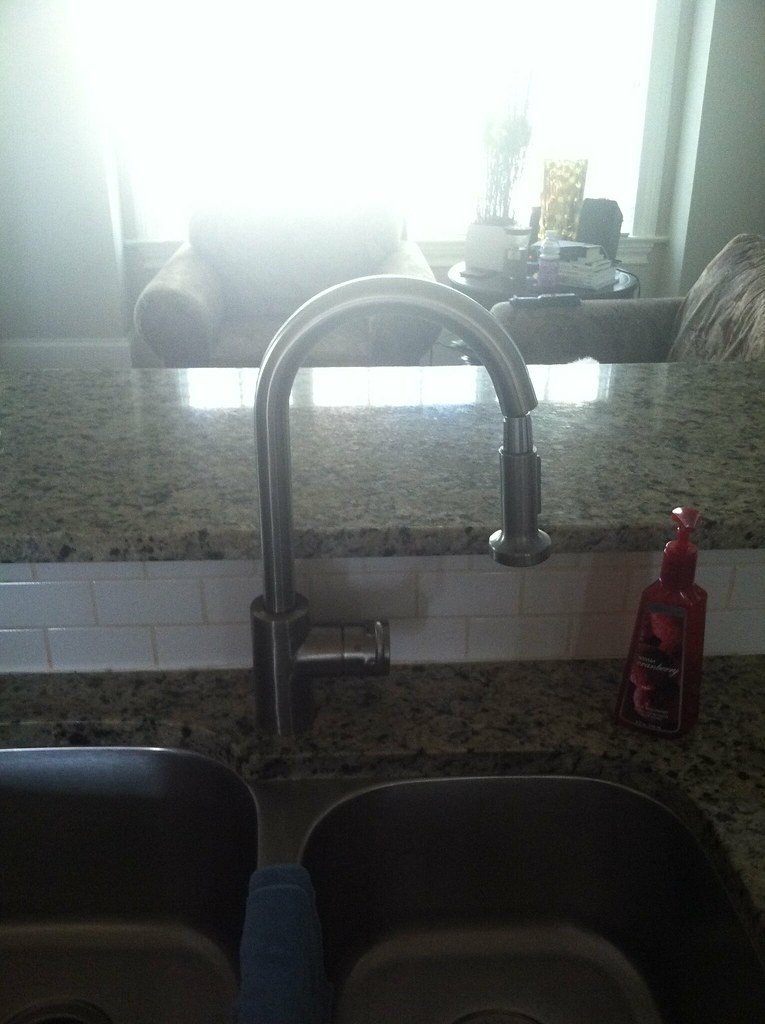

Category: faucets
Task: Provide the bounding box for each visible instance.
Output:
[245,273,554,738]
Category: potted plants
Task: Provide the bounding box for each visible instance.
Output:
[464,102,530,264]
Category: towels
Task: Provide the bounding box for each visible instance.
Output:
[234,862,335,1024]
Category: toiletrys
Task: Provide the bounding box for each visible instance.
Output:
[615,506,708,742]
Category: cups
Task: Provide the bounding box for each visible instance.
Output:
[504,226,532,281]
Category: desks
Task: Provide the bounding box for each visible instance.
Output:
[449,256,640,365]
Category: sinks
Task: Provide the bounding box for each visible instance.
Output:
[261,750,765,1024]
[0,739,268,1024]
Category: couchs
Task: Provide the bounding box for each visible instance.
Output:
[131,166,765,372]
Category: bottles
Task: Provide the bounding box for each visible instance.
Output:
[539,230,561,293]
